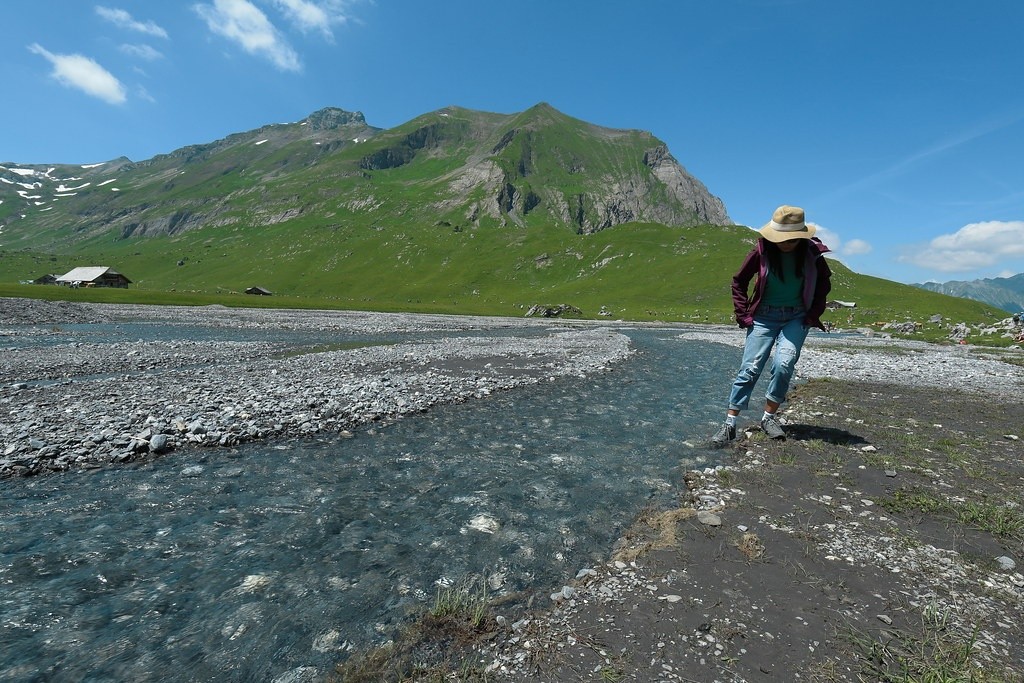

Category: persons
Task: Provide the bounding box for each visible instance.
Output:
[75,282,79,290]
[68,280,73,290]
[713,205,833,440]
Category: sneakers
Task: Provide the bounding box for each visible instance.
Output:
[710,422,736,445]
[761,418,786,440]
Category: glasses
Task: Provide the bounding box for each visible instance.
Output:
[787,239,797,243]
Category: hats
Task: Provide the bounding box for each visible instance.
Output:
[759,205,816,243]
